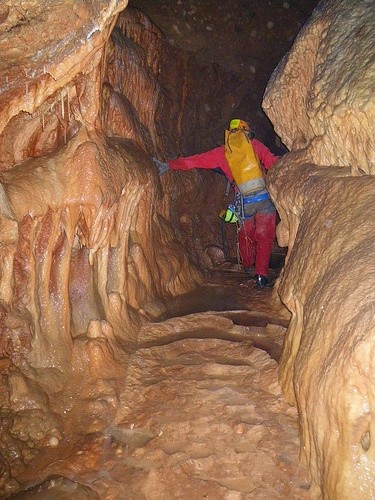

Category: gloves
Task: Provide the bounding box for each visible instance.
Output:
[152,158,170,173]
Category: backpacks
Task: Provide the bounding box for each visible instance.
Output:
[224,129,265,196]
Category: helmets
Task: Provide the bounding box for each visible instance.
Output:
[229,119,250,135]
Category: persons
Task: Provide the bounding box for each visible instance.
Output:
[153,118,281,290]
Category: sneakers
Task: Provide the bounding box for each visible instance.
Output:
[256,274,266,288]
[245,267,253,274]
